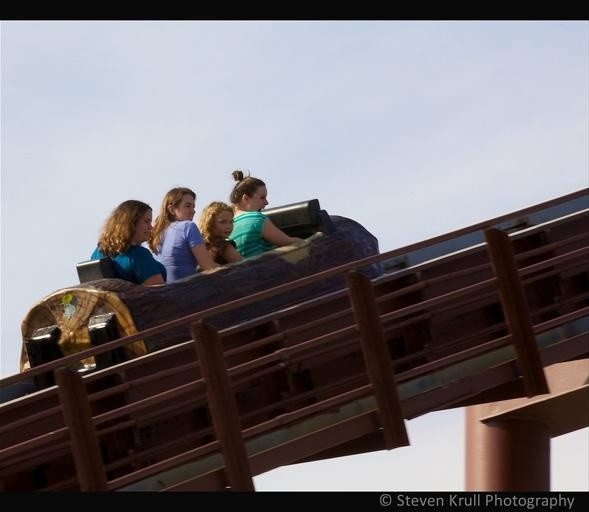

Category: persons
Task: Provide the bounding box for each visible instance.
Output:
[145,187,223,285]
[196,201,244,273]
[225,169,307,260]
[90,199,168,287]
